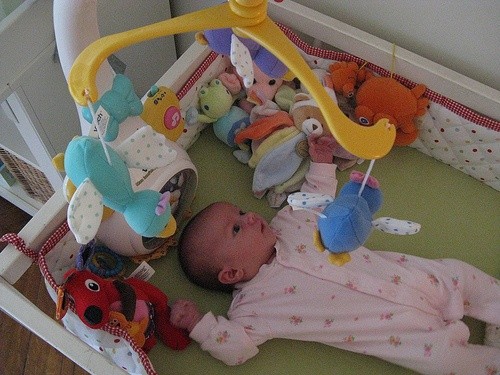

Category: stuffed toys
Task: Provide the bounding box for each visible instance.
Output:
[51,32,430,276]
[56,268,192,350]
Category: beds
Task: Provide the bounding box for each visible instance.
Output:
[0,0,500,375]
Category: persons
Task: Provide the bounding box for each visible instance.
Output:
[170,135,500,375]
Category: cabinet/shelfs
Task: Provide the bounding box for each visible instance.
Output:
[0,0,178,219]
